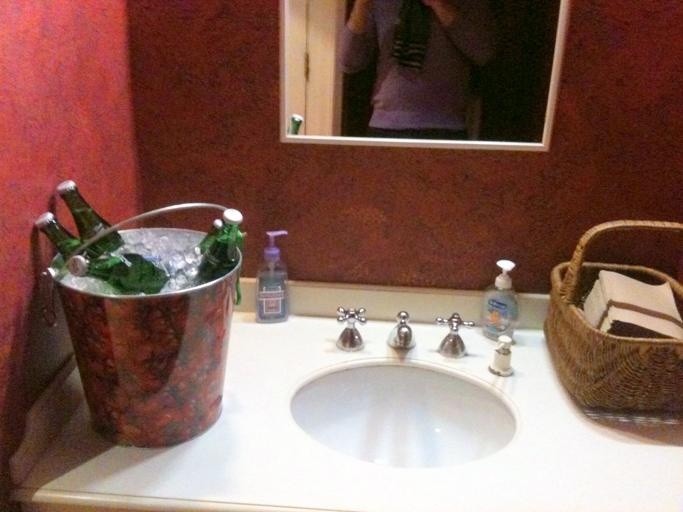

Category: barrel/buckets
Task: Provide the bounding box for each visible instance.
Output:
[39,201,244,449]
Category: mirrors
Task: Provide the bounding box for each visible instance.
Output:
[278,0,570,154]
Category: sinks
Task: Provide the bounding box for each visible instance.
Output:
[290,356,520,472]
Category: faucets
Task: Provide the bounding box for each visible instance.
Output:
[387,311,415,350]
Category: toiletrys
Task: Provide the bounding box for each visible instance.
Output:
[481,259,520,342]
[255,230,289,322]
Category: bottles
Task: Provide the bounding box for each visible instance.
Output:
[288,114,303,134]
[34,180,243,295]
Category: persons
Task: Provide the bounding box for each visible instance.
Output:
[333,1,497,139]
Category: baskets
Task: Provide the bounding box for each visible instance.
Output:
[543,219,683,414]
